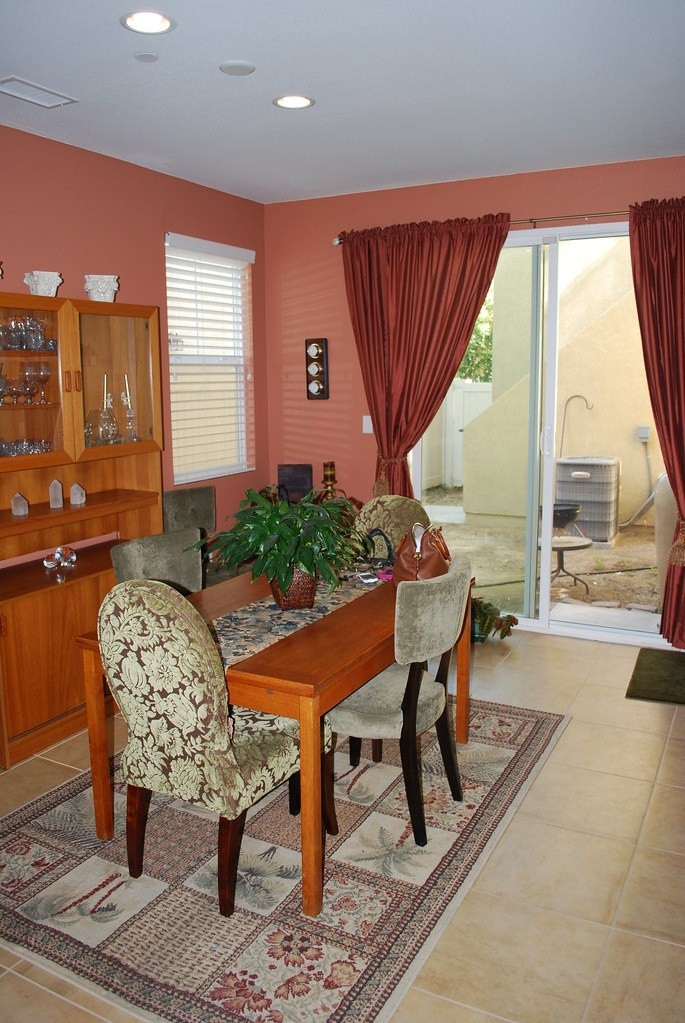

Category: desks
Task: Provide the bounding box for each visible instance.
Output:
[537,534,600,595]
[77,564,477,917]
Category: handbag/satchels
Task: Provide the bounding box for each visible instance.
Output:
[393,523,451,587]
[352,529,393,566]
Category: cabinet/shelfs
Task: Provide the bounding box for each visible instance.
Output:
[0,291,166,772]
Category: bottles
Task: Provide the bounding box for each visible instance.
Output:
[85,407,118,445]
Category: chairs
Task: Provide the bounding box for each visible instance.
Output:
[110,528,210,606]
[163,486,254,585]
[96,575,337,917]
[349,495,434,562]
[320,567,477,847]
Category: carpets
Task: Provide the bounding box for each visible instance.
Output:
[626,646,685,706]
[0,682,572,1023]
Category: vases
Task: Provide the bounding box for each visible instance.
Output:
[472,617,492,643]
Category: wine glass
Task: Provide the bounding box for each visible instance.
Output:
[0,361,51,407]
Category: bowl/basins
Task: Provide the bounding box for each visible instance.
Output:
[539,504,581,528]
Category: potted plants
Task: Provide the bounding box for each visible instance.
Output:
[180,486,373,611]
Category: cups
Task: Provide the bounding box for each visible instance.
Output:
[0,313,56,352]
[84,274,118,303]
[22,271,63,297]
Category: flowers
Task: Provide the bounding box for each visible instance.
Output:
[471,577,520,640]
